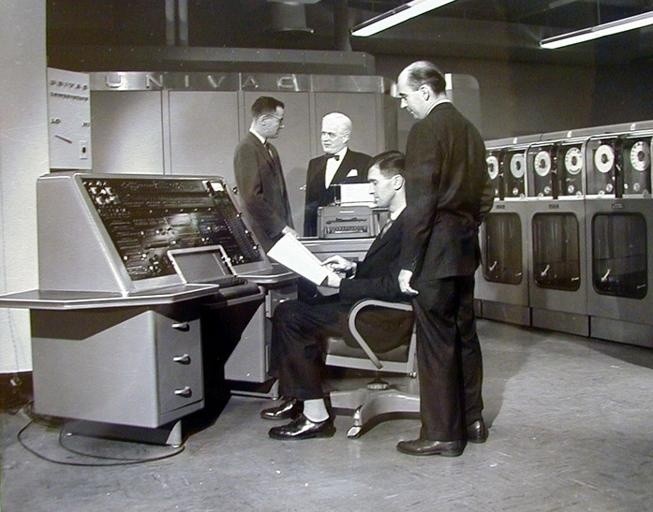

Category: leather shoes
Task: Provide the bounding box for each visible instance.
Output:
[397,420,485,457]
[260,399,303,420]
[269,408,336,440]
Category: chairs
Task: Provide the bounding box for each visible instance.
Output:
[321,297,421,440]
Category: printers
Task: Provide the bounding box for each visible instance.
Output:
[317,203,376,240]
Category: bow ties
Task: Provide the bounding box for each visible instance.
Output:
[325,153,339,160]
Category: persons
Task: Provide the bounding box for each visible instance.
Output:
[301,112,374,239]
[392,60,494,456]
[232,97,295,253]
[259,151,408,442]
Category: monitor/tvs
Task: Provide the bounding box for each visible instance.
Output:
[166,244,225,282]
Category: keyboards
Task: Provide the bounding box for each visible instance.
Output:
[198,277,246,288]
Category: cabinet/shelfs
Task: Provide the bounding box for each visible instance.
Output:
[221,285,300,385]
[32,312,203,451]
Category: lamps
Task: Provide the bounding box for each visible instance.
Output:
[539,0,653,56]
[349,1,455,42]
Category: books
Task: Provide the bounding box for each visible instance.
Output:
[262,233,334,287]
